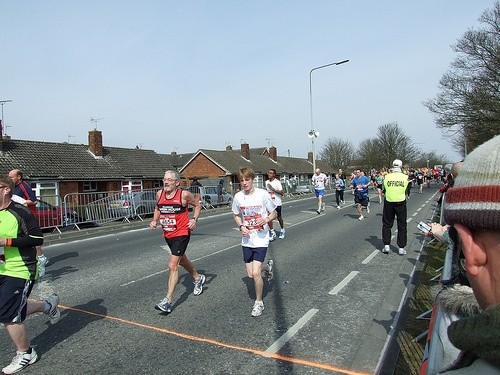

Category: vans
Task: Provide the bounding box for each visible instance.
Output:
[445,164,453,171]
[186,185,234,209]
[434,165,443,170]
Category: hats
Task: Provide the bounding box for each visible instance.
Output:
[393,159,402,168]
[443,135,500,231]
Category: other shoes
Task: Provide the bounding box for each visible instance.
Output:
[399,250,406,254]
[316,210,320,214]
[322,207,325,210]
[382,247,390,252]
[358,216,364,220]
[337,206,340,209]
[343,201,345,204]
[367,209,370,213]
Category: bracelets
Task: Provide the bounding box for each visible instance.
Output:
[6,238,12,246]
[275,189,276,191]
[193,217,198,222]
[239,225,243,231]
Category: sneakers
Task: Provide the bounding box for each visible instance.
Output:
[251,300,264,316]
[269,232,276,241]
[154,298,172,312]
[44,293,60,325]
[38,257,49,277]
[279,229,286,239]
[267,260,273,281]
[2,348,38,374]
[193,274,205,295]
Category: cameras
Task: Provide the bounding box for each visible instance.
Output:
[416,221,431,234]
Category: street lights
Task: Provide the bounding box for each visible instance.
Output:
[427,159,429,168]
[308,59,350,176]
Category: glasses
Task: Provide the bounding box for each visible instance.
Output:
[162,178,178,183]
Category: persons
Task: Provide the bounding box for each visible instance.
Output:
[334,174,344,209]
[349,165,449,211]
[427,137,500,375]
[0,174,61,374]
[312,168,328,214]
[8,169,49,277]
[382,159,409,255]
[217,179,224,208]
[189,176,203,211]
[149,169,205,313]
[232,167,278,316]
[335,168,345,205]
[264,169,286,242]
[351,169,371,221]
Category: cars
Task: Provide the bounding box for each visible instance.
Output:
[27,199,85,232]
[106,191,159,220]
[295,181,316,193]
